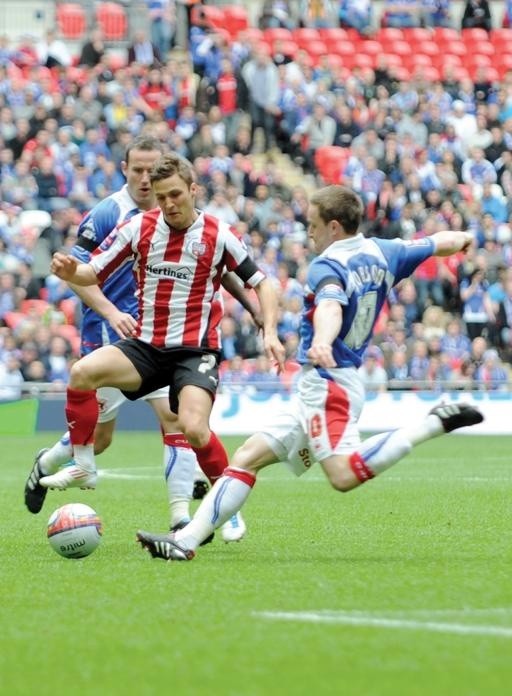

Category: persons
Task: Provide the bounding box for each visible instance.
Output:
[135,182,486,561]
[37,150,287,545]
[0,0,512,393]
[23,132,216,544]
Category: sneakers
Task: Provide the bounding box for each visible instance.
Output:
[220,511,244,542]
[192,472,212,496]
[431,402,485,434]
[25,450,50,514]
[137,530,196,561]
[38,463,99,491]
[172,519,213,547]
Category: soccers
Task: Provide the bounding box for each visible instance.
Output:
[48,503,103,559]
